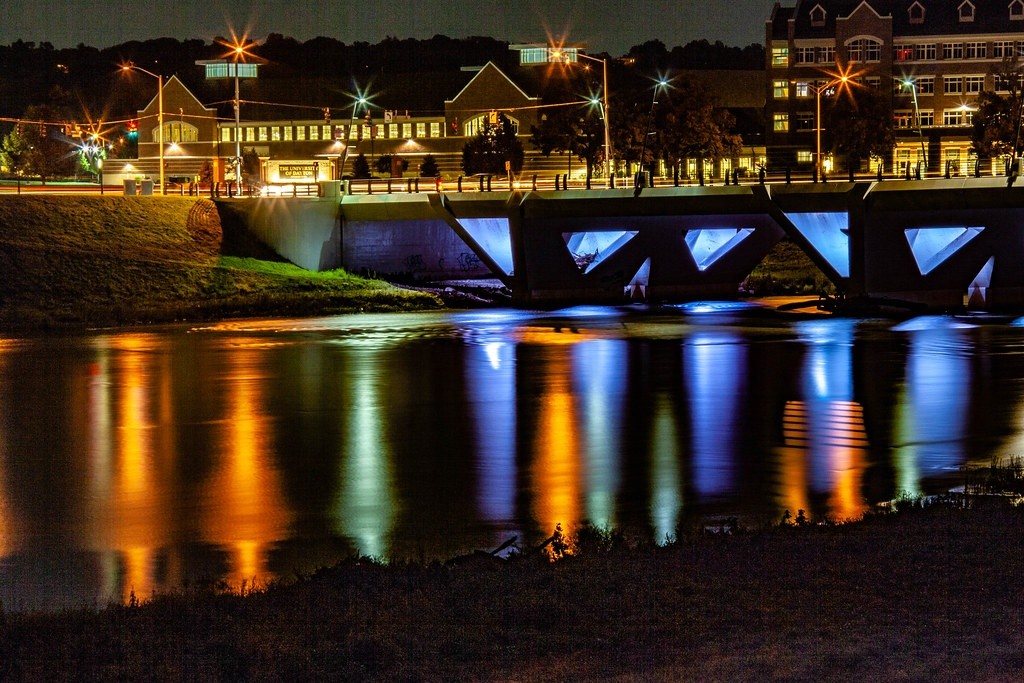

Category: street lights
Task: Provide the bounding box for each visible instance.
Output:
[591,99,619,178]
[234,46,243,197]
[123,65,165,194]
[636,80,670,188]
[552,50,611,189]
[340,99,368,180]
[816,76,847,183]
[903,81,927,167]
[93,134,106,160]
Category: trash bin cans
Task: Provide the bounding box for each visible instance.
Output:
[141,180,153,194]
[321,180,341,194]
[391,158,402,177]
[637,171,650,187]
[123,179,137,195]
